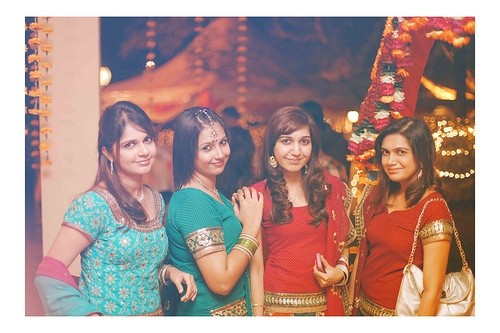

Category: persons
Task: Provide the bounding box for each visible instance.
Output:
[36,100,453,316]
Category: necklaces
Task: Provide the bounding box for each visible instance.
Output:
[136,185,145,202]
[196,179,220,199]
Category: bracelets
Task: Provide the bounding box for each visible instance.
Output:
[159,263,172,287]
[334,264,349,287]
[233,234,260,259]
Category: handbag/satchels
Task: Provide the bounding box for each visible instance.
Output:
[395,198,475,316]
